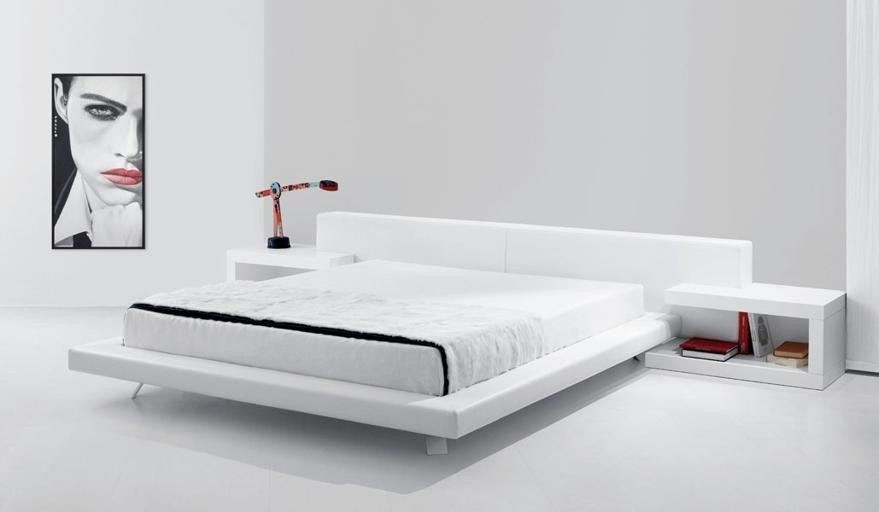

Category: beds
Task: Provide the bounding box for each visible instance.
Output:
[61,206,755,457]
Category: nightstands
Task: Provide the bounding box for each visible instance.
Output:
[641,274,849,393]
[224,243,323,284]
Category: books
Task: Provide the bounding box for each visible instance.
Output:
[678,310,809,373]
